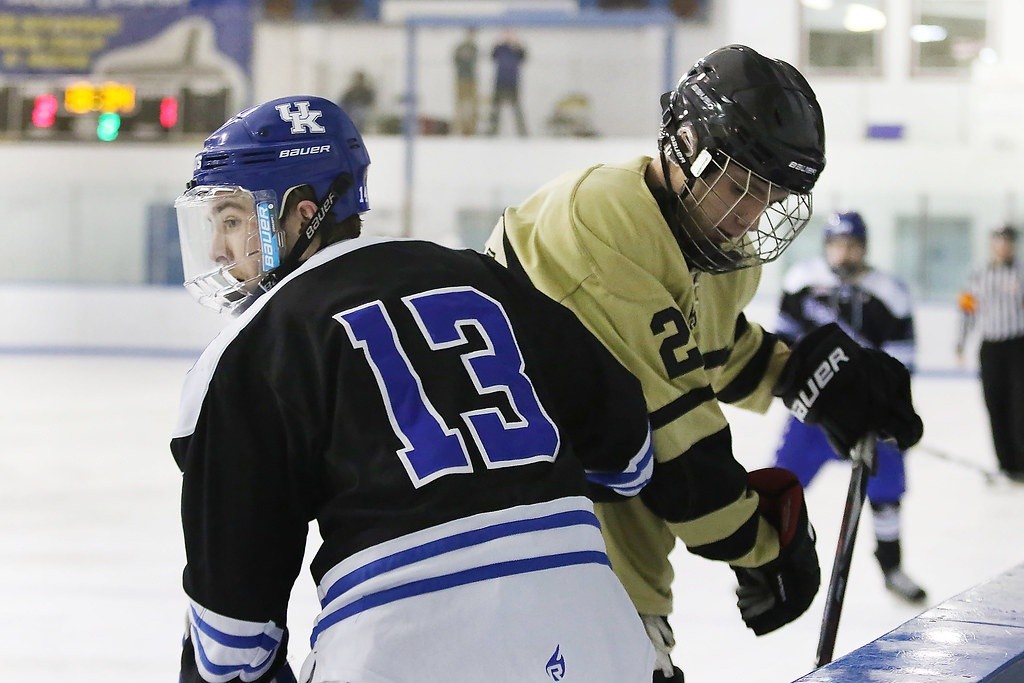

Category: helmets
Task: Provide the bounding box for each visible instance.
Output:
[824,209,867,278]
[185,95,371,314]
[658,44,826,275]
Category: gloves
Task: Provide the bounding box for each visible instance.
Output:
[771,322,924,477]
[730,467,821,636]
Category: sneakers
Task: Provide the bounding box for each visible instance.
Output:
[884,571,926,606]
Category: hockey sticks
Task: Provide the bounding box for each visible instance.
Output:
[815,420,882,669]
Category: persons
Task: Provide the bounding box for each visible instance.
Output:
[340,72,375,132]
[453,26,479,134]
[954,226,1024,483]
[768,212,927,605]
[484,27,528,136]
[484,44,924,683]
[167,94,655,683]
